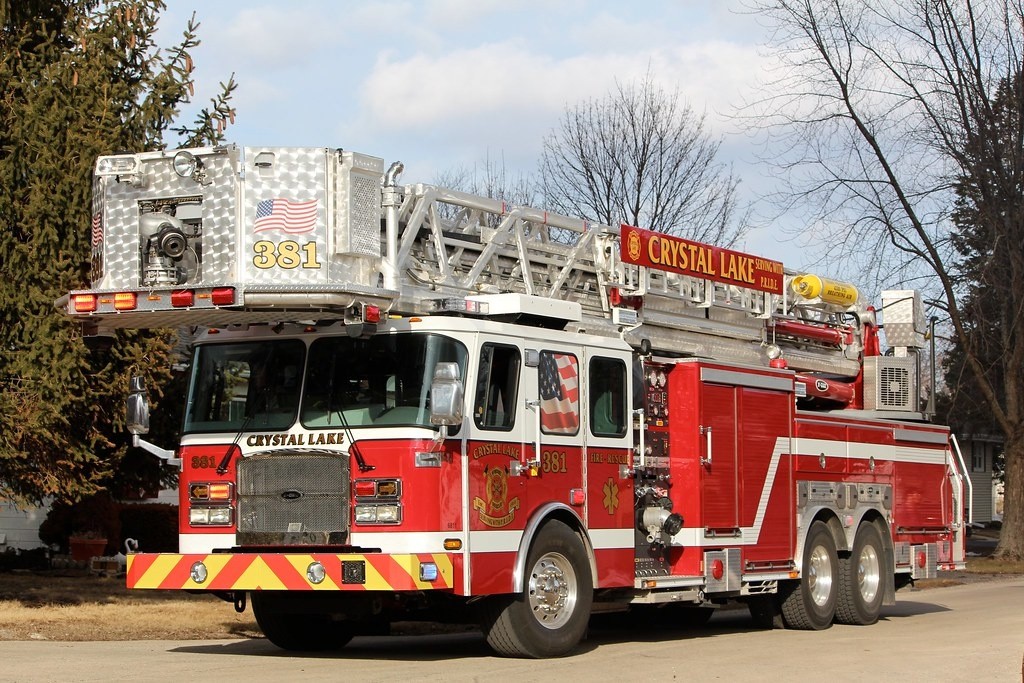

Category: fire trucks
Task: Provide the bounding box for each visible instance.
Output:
[53,141,972,659]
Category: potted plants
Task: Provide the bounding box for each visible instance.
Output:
[70,531,108,569]
